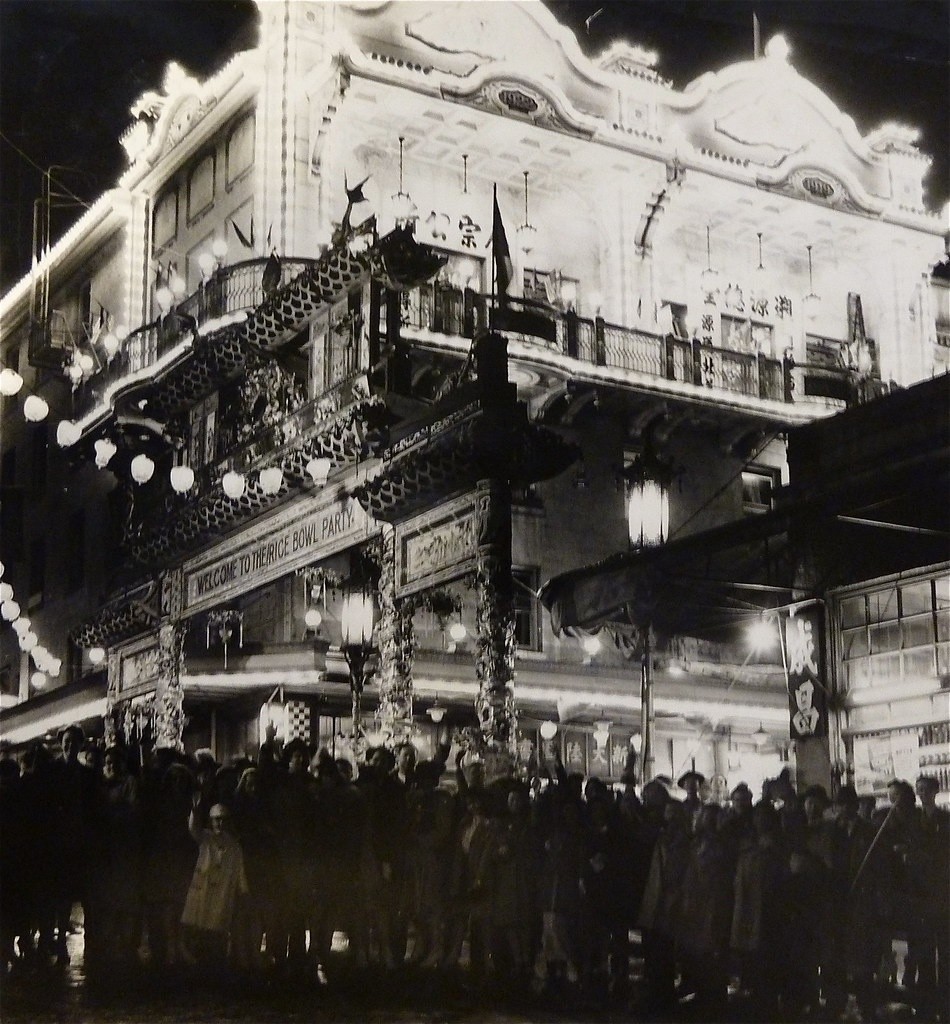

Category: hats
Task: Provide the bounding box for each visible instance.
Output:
[1,724,950,821]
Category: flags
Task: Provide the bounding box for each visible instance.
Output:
[492,195,517,314]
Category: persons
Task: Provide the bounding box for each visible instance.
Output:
[0,699,950,1024]
